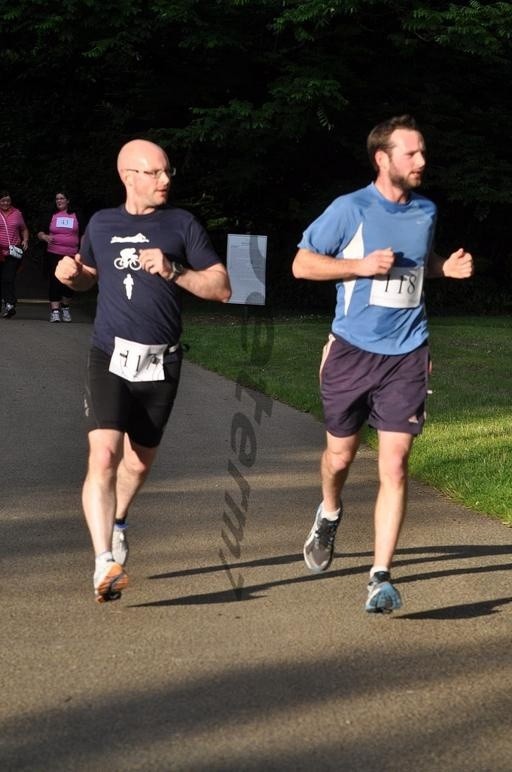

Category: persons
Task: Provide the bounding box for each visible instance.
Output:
[33,191,84,324]
[292,115,475,614]
[1,191,29,319]
[54,139,232,603]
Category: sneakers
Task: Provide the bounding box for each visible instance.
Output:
[62,307,72,322]
[49,309,60,322]
[2,304,16,319]
[366,571,402,617]
[304,501,343,572]
[92,523,130,603]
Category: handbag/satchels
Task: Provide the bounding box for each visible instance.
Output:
[8,246,23,258]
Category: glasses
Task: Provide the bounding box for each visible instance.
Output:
[125,165,177,177]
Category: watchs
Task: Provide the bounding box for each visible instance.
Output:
[166,261,185,282]
